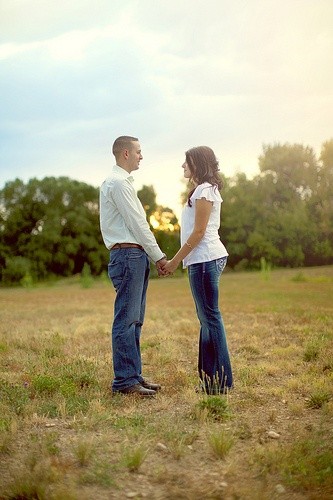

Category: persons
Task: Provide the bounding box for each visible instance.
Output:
[98,136,172,399]
[159,146,234,395]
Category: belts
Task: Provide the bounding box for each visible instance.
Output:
[111,243,144,251]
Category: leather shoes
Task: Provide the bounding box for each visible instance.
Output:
[141,380,162,391]
[118,382,157,397]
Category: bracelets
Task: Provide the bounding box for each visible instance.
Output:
[185,241,194,250]
